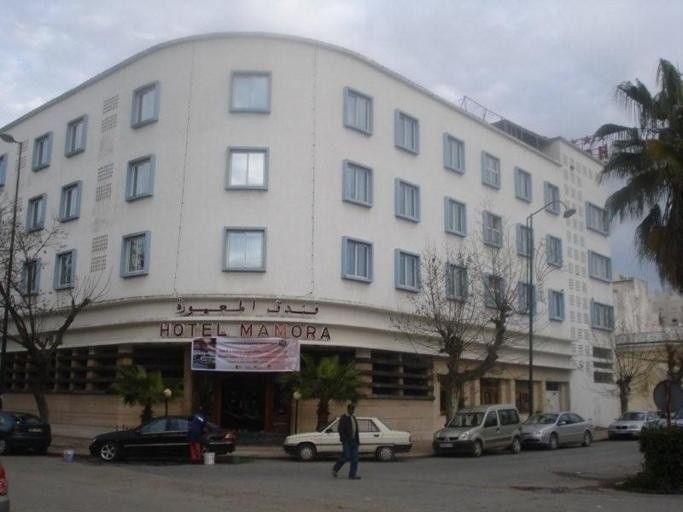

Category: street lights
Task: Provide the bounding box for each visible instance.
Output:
[1,131,24,377]
[525,198,577,414]
[293,390,303,435]
[161,388,173,416]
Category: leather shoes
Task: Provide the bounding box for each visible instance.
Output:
[349,474,361,479]
[332,465,338,477]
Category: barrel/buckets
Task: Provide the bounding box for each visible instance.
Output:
[203,452,216,465]
[63,449,76,463]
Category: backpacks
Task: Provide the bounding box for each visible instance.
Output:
[337,420,342,441]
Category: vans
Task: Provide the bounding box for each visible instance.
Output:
[431,402,524,459]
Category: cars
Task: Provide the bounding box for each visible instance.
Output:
[606,410,660,440]
[1,411,53,456]
[283,414,413,461]
[88,414,237,463]
[520,410,595,450]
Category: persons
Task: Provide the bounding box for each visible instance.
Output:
[186,403,205,464]
[331,402,362,480]
